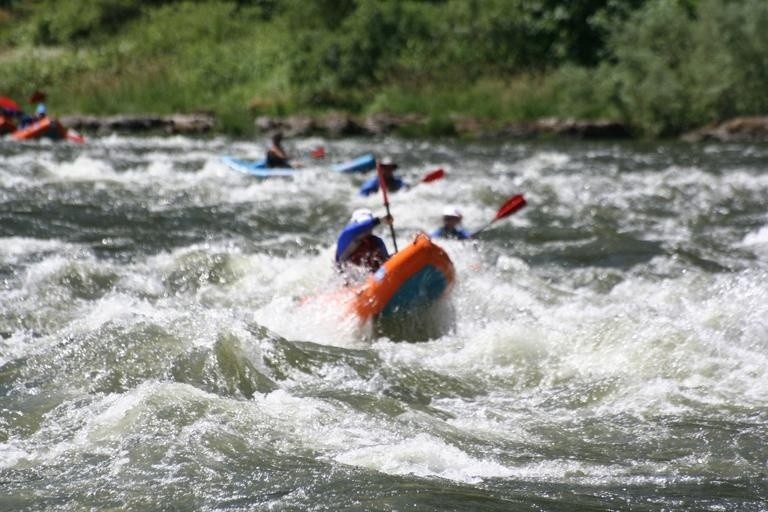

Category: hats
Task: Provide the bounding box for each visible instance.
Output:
[351,208,373,225]
[380,156,397,171]
[442,208,461,218]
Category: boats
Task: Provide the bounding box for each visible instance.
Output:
[0,116,85,145]
[223,154,376,183]
[299,231,455,326]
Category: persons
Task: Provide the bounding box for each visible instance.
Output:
[428,209,474,241]
[264,129,295,169]
[19,103,46,130]
[357,155,412,200]
[335,206,393,295]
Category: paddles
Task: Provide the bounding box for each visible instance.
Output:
[470,196,527,238]
[410,169,444,188]
[376,159,398,251]
